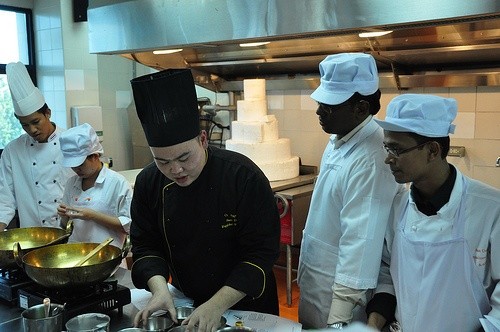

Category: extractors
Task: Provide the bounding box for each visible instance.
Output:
[86,0,500,92]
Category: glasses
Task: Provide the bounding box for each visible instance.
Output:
[318,102,337,114]
[382,139,432,159]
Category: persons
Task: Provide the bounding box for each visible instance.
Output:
[57,122,134,270]
[129,97,282,332]
[295,52,410,332]
[0,87,78,230]
[361,93,500,332]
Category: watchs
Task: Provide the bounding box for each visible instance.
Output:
[326,321,351,330]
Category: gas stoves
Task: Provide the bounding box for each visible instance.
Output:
[0,268,33,307]
[17,279,131,319]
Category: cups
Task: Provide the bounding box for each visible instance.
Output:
[21,303,65,332]
[66,313,110,332]
[118,306,257,332]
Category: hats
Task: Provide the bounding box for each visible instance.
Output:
[6,61,45,117]
[59,123,104,168]
[131,68,203,147]
[374,94,457,137]
[310,52,379,105]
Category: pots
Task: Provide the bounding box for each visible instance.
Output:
[0,220,74,267]
[13,243,130,290]
